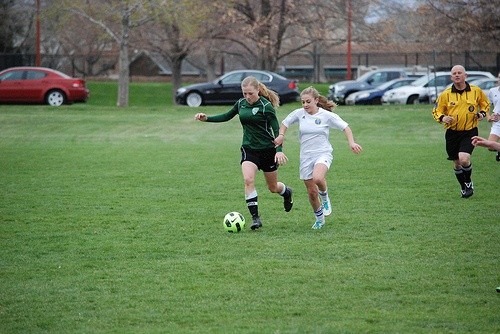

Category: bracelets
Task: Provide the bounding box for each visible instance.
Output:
[482,113,485,117]
[280,134,284,136]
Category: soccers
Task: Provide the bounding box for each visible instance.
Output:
[223,211,246,234]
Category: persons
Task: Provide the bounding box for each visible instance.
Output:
[471,136,500,152]
[486,73,500,161]
[194,76,293,230]
[273,87,363,229]
[432,65,489,198]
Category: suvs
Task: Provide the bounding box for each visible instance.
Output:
[327,68,432,103]
[381,71,496,105]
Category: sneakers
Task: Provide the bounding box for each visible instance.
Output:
[322,198,332,216]
[250,218,262,230]
[284,186,292,212]
[465,182,473,197]
[461,187,466,197]
[312,219,325,229]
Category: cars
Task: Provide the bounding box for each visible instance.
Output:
[345,78,419,105]
[430,77,499,106]
[175,70,300,107]
[0,67,90,106]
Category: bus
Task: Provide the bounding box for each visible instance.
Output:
[276,64,379,86]
